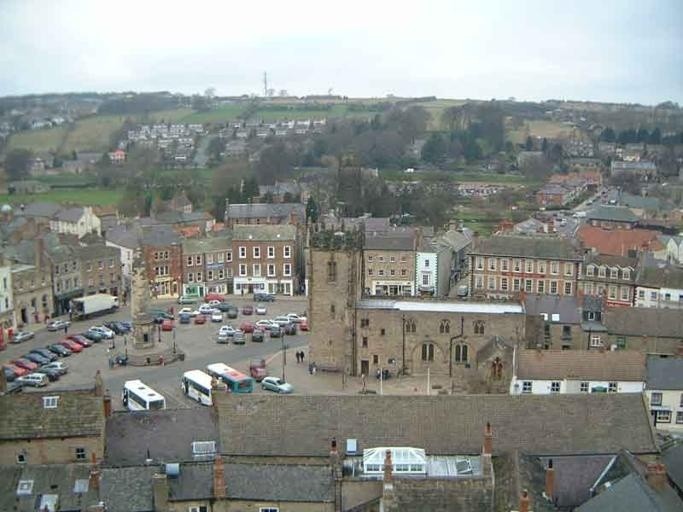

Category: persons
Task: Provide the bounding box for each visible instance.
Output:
[300,351,304,364]
[65,325,67,335]
[147,356,150,366]
[376,369,389,384]
[296,351,300,364]
[70,312,72,321]
[159,355,164,365]
[241,289,243,297]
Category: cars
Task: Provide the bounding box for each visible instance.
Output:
[261,376,294,393]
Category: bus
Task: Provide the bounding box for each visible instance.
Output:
[181,369,228,408]
[121,379,166,410]
[206,362,255,393]
